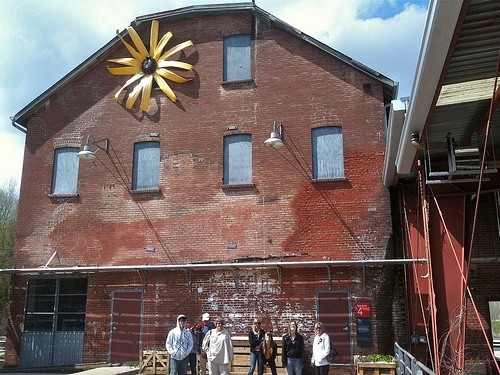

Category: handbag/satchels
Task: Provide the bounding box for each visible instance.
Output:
[326,344,338,362]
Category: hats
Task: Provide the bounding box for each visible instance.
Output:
[201,313,210,321]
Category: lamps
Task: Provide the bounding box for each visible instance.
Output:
[263,121,284,148]
[76,134,109,161]
[410,131,427,152]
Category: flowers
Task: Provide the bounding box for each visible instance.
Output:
[107,20,194,113]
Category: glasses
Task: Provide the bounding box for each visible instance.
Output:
[217,323,224,324]
[255,322,260,325]
[317,327,322,329]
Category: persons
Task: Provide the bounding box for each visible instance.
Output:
[310,322,330,375]
[166,313,305,375]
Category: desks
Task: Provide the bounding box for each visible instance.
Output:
[356,361,397,375]
